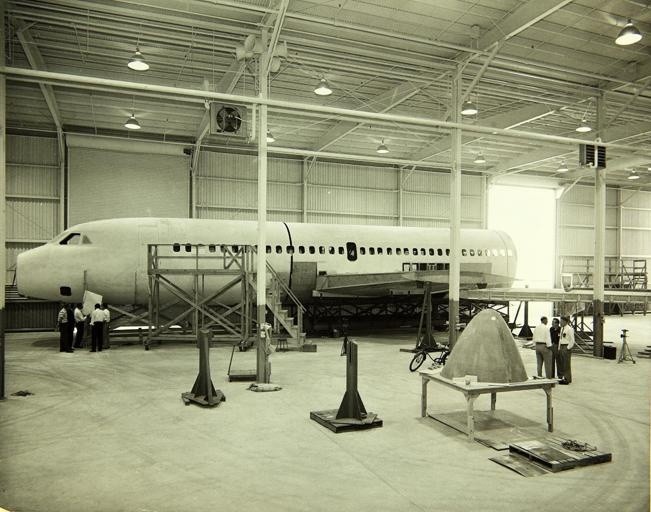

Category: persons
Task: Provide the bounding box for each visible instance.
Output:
[52,302,112,353]
[532,316,575,385]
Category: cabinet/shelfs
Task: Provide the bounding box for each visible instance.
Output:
[559,258,648,315]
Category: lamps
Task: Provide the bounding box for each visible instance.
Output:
[314,78,333,95]
[461,100,479,116]
[377,138,389,153]
[128,47,149,71]
[614,19,644,45]
[266,130,275,142]
[474,153,486,164]
[125,114,140,129]
[628,169,640,180]
[575,118,592,133]
[556,161,568,172]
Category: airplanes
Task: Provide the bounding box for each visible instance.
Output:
[12,215,518,356]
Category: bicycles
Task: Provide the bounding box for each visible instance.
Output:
[406,336,454,372]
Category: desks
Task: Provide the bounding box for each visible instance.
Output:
[418,369,558,443]
